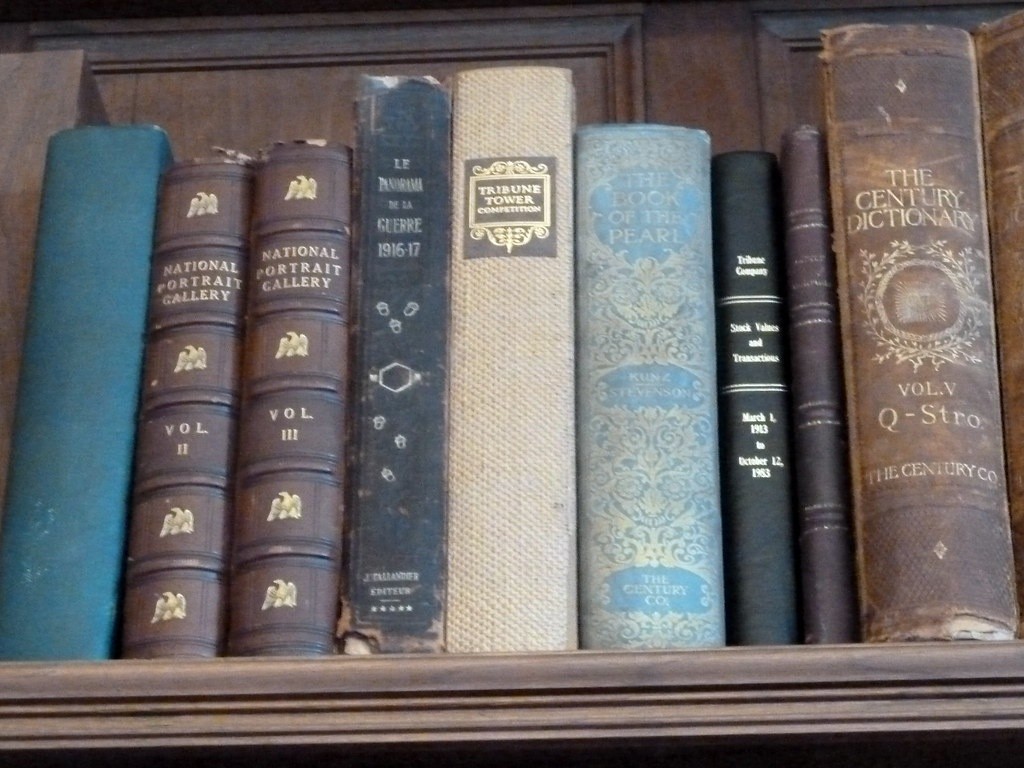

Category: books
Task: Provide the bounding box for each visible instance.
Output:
[1,8,1024,666]
